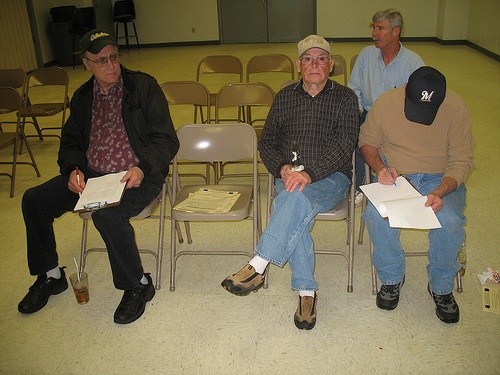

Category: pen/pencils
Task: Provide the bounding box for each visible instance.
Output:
[76,167,81,197]
[376,147,397,186]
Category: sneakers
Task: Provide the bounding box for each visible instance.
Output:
[376,275,405,310]
[18,266,68,313]
[294,291,317,330]
[427,283,459,323]
[114,273,155,324]
[221,262,266,296]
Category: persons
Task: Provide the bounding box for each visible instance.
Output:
[345,7,425,207]
[358,66,475,324]
[220,34,359,330]
[16,28,179,325]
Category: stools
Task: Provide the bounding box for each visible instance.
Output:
[68,6,97,70]
[113,0,140,50]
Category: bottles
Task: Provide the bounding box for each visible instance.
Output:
[460,232,467,276]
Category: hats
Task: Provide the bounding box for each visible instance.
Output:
[79,29,118,54]
[404,66,446,126]
[297,34,331,58]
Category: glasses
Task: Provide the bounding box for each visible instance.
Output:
[368,24,385,32]
[299,56,330,65]
[83,53,119,67]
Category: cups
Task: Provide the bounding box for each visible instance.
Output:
[69,272,90,305]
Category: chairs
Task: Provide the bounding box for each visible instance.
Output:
[358,162,463,294]
[167,122,257,294]
[193,54,243,124]
[215,81,275,186]
[297,55,349,86]
[0,68,26,154]
[245,51,293,128]
[20,67,71,155]
[158,80,210,186]
[0,86,41,198]
[79,173,183,290]
[263,149,357,292]
